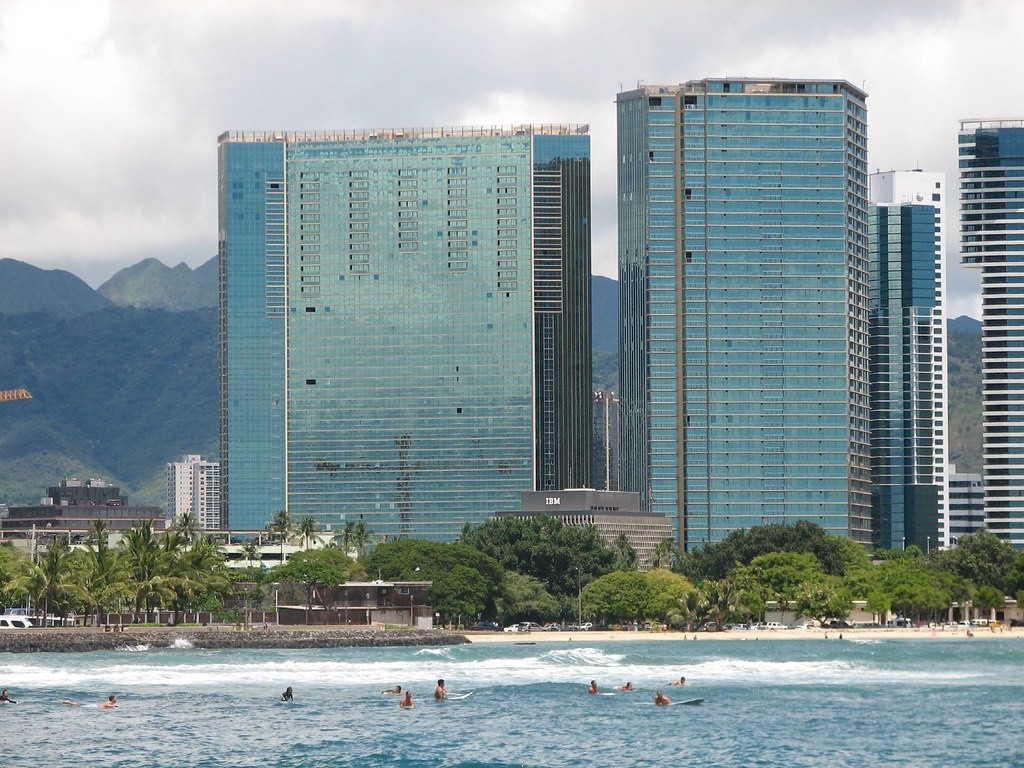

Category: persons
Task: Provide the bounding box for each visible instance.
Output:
[588,677,685,705]
[663,623,667,634]
[0,689,119,708]
[628,620,638,632]
[280,687,293,700]
[528,622,532,634]
[825,624,1012,640]
[380,679,447,707]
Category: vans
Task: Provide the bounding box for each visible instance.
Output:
[1,614,33,629]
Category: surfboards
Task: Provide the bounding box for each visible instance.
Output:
[596,692,617,697]
[450,691,473,700]
[669,698,704,706]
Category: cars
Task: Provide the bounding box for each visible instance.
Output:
[501,621,667,632]
[681,620,788,632]
